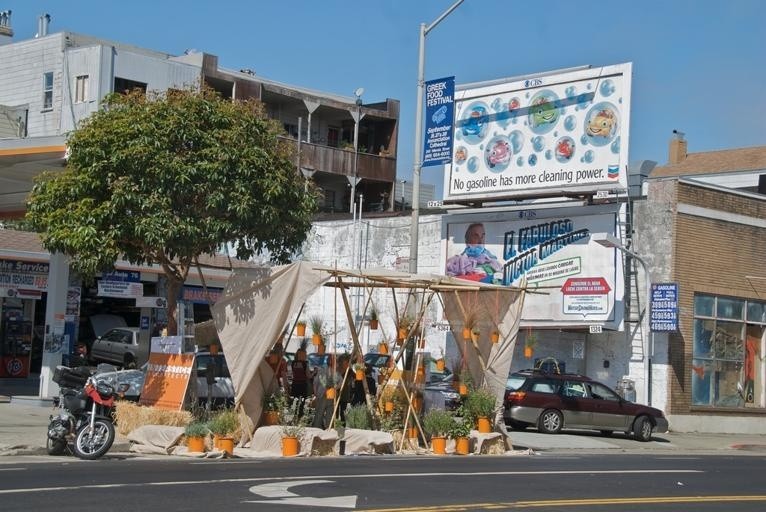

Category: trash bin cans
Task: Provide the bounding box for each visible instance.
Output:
[424,381,460,418]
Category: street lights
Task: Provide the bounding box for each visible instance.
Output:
[592,232,652,407]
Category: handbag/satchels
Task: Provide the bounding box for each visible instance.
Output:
[53,364,91,390]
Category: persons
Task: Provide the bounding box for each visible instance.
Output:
[336,363,353,429]
[311,369,345,430]
[446,224,503,276]
[350,378,376,408]
[292,360,318,424]
[264,352,288,388]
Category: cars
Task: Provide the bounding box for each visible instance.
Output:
[502,368,668,442]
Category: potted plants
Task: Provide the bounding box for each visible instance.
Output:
[207,414,236,454]
[270,307,500,412]
[470,390,497,432]
[423,410,451,453]
[407,416,418,438]
[185,423,205,453]
[209,336,219,355]
[451,421,472,456]
[524,338,534,359]
[262,393,278,424]
[281,421,303,454]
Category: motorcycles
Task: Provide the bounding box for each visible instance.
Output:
[47,363,130,459]
